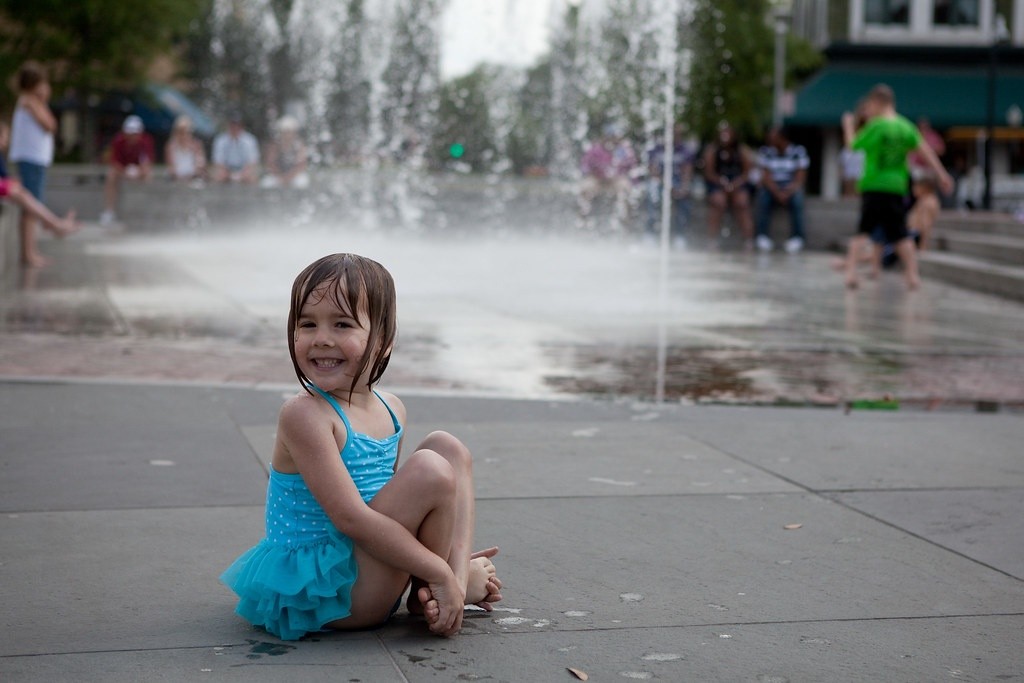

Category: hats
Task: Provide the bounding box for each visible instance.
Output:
[122,115,144,135]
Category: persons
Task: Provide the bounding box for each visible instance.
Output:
[840,82,955,298]
[542,117,809,255]
[0,61,310,270]
[220,253,503,639]
